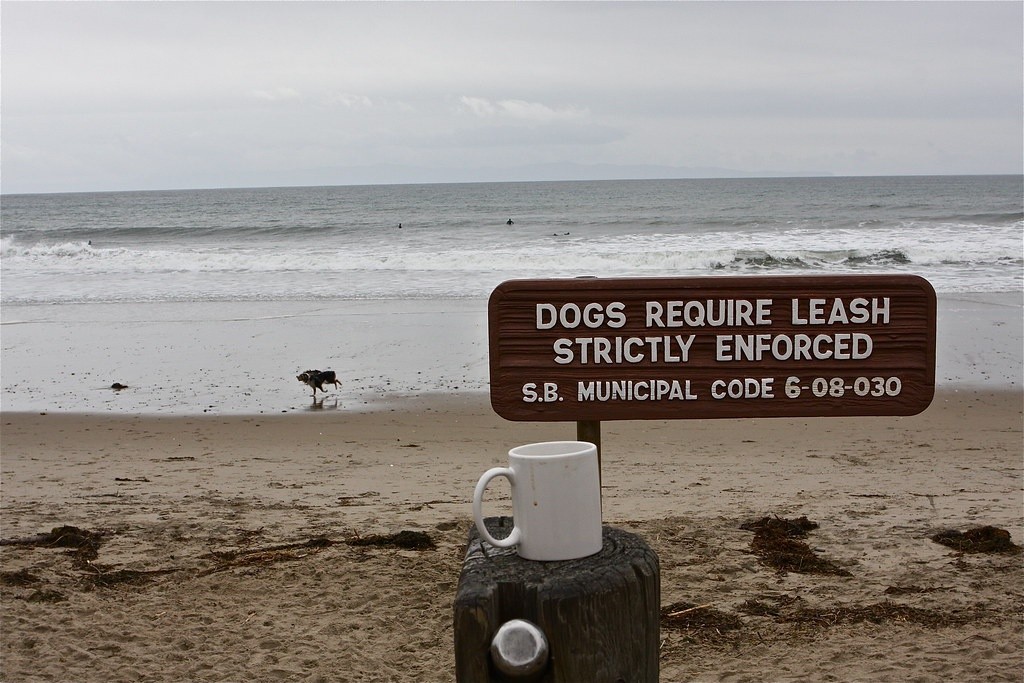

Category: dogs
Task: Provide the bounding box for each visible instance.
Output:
[296,369,343,396]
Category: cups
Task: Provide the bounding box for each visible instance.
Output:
[472,440,603,561]
